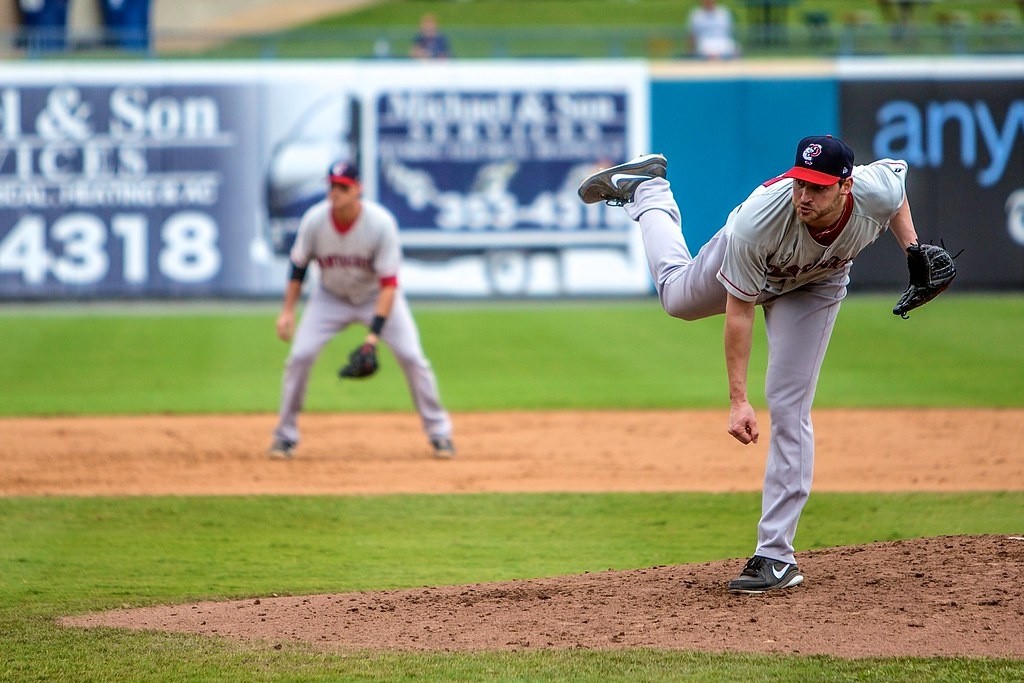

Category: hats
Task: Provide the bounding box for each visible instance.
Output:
[784,135,855,186]
[330,161,359,187]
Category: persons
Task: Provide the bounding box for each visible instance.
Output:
[683,1,741,61]
[270,158,457,460]
[578,133,956,595]
[409,14,453,58]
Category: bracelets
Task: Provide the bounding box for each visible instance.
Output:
[370,315,386,337]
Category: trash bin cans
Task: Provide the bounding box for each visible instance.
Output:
[99,0,150,52]
[12,0,67,52]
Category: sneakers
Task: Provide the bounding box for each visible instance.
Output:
[434,437,455,459]
[270,442,295,460]
[579,155,668,208]
[729,556,804,592]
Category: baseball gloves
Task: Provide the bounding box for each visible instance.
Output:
[892,242,957,315]
[337,343,378,378]
